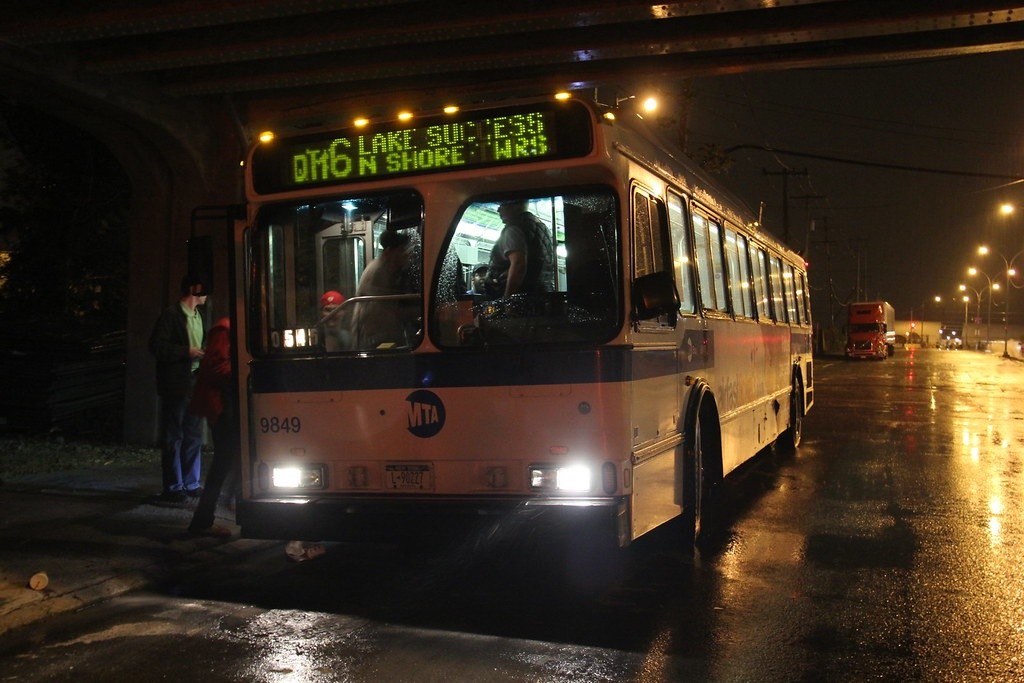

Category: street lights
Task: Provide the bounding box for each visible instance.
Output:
[958,282,1001,351]
[967,265,1016,350]
[978,244,1024,357]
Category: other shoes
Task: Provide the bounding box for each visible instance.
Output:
[188,486,204,498]
[161,490,189,504]
[187,523,231,539]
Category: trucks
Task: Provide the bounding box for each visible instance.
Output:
[841,301,897,361]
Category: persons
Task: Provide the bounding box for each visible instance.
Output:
[467,264,488,293]
[350,229,412,348]
[148,273,211,508]
[485,198,553,303]
[320,291,351,350]
[392,220,468,323]
[189,315,240,537]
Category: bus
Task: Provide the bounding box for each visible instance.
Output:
[184,80,816,567]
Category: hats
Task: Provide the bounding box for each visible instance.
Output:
[473,264,488,274]
[379,229,412,249]
[320,290,344,309]
